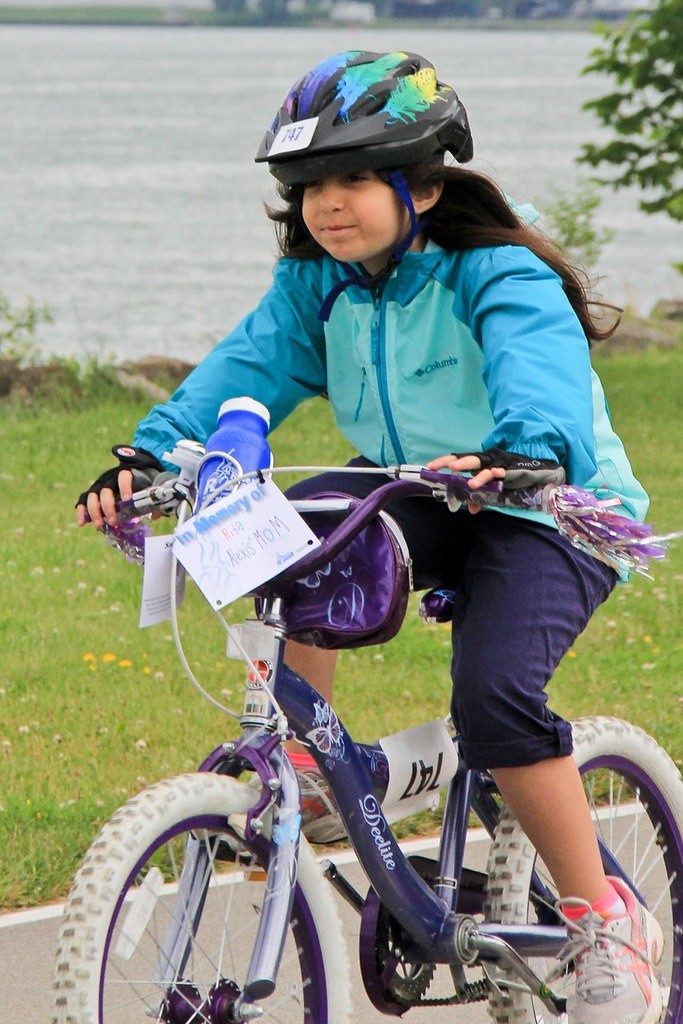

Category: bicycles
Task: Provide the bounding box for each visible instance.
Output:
[46,439,683,1023]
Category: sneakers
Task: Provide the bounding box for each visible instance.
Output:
[568,874,667,1024]
[206,768,354,861]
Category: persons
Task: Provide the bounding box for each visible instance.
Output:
[74,47,672,1024]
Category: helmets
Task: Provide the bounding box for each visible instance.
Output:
[256,51,473,186]
